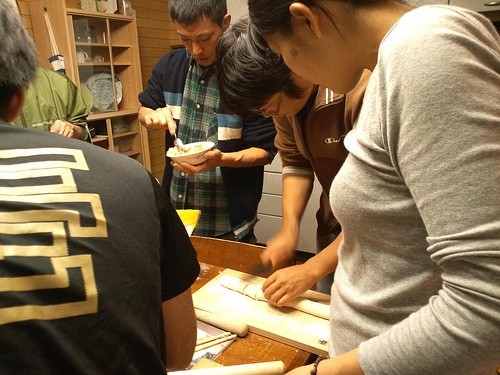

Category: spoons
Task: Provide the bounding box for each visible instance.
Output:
[173,130,185,153]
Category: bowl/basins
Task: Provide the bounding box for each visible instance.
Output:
[165,141,215,166]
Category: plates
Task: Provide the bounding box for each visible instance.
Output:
[84,70,123,111]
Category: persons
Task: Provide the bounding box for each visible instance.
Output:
[247,0,500,375]
[138,0,277,245]
[214,15,373,308]
[9,64,94,141]
[0,0,201,375]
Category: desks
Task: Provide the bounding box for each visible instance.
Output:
[166,236,317,375]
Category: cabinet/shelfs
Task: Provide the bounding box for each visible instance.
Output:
[29,0,151,174]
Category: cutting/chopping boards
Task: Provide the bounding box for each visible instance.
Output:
[192,268,332,359]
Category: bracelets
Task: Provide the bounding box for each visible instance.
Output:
[309,353,327,375]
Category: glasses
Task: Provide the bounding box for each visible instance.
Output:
[254,91,282,116]
